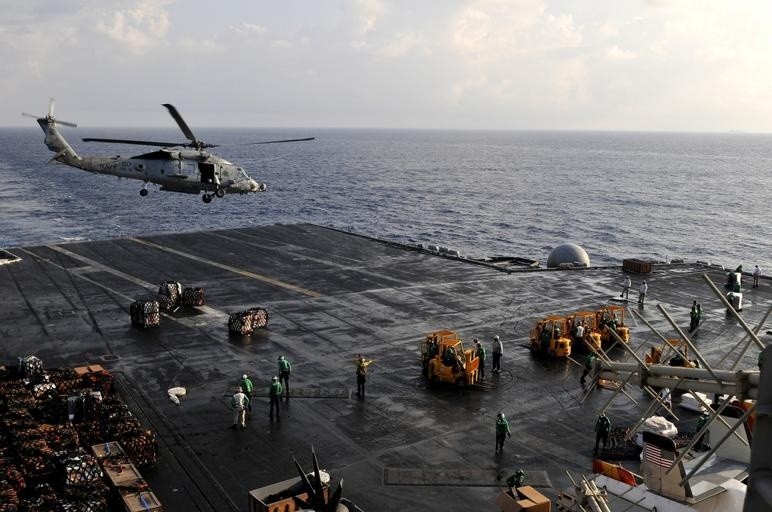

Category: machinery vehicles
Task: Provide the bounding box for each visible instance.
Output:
[644,336,704,382]
[418,327,480,389]
[530,303,633,360]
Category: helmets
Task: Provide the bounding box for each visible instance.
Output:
[272,375,279,381]
[496,412,506,419]
[277,355,285,360]
[242,373,248,379]
[359,357,367,362]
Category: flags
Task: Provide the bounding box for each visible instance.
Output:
[645,443,674,468]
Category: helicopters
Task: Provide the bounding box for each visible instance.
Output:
[21,95,316,206]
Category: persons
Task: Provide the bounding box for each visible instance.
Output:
[507,469,525,489]
[278,355,292,401]
[620,275,632,299]
[539,318,618,385]
[269,376,283,424]
[238,373,253,412]
[230,386,250,430]
[752,265,761,288]
[356,358,373,401]
[736,265,742,273]
[687,301,703,333]
[429,335,503,381]
[695,411,710,442]
[656,387,672,419]
[638,280,647,303]
[496,413,511,451]
[592,413,611,451]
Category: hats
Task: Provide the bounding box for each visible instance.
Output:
[473,337,479,342]
[235,386,244,393]
[493,335,500,339]
